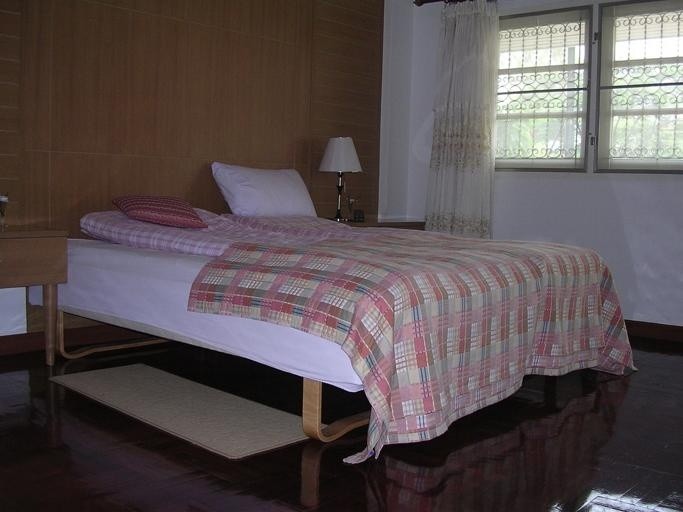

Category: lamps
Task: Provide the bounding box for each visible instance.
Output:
[317,137,363,221]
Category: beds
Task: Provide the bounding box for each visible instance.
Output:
[57,215,638,445]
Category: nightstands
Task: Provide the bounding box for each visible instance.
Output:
[346,220,427,230]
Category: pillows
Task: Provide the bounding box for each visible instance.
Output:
[112,163,317,229]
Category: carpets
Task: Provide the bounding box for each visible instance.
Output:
[47,364,327,460]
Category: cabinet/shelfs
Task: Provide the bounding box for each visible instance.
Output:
[0,230,69,367]
